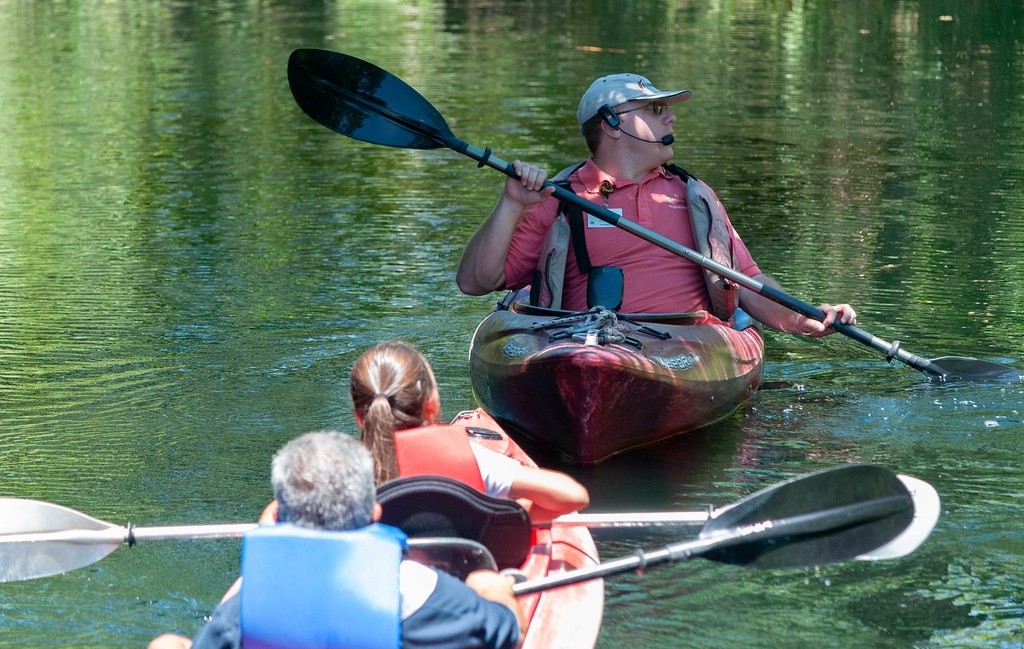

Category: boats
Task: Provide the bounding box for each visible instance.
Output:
[209,407,608,649]
[465,280,768,472]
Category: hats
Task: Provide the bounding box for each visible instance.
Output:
[577,72,693,129]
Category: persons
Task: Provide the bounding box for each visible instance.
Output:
[147,428,527,649]
[260,340,591,524]
[456,72,858,339]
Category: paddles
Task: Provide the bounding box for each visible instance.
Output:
[512,463,915,598]
[285,46,1013,376]
[0,474,943,589]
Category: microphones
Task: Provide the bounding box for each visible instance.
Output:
[598,104,674,145]
